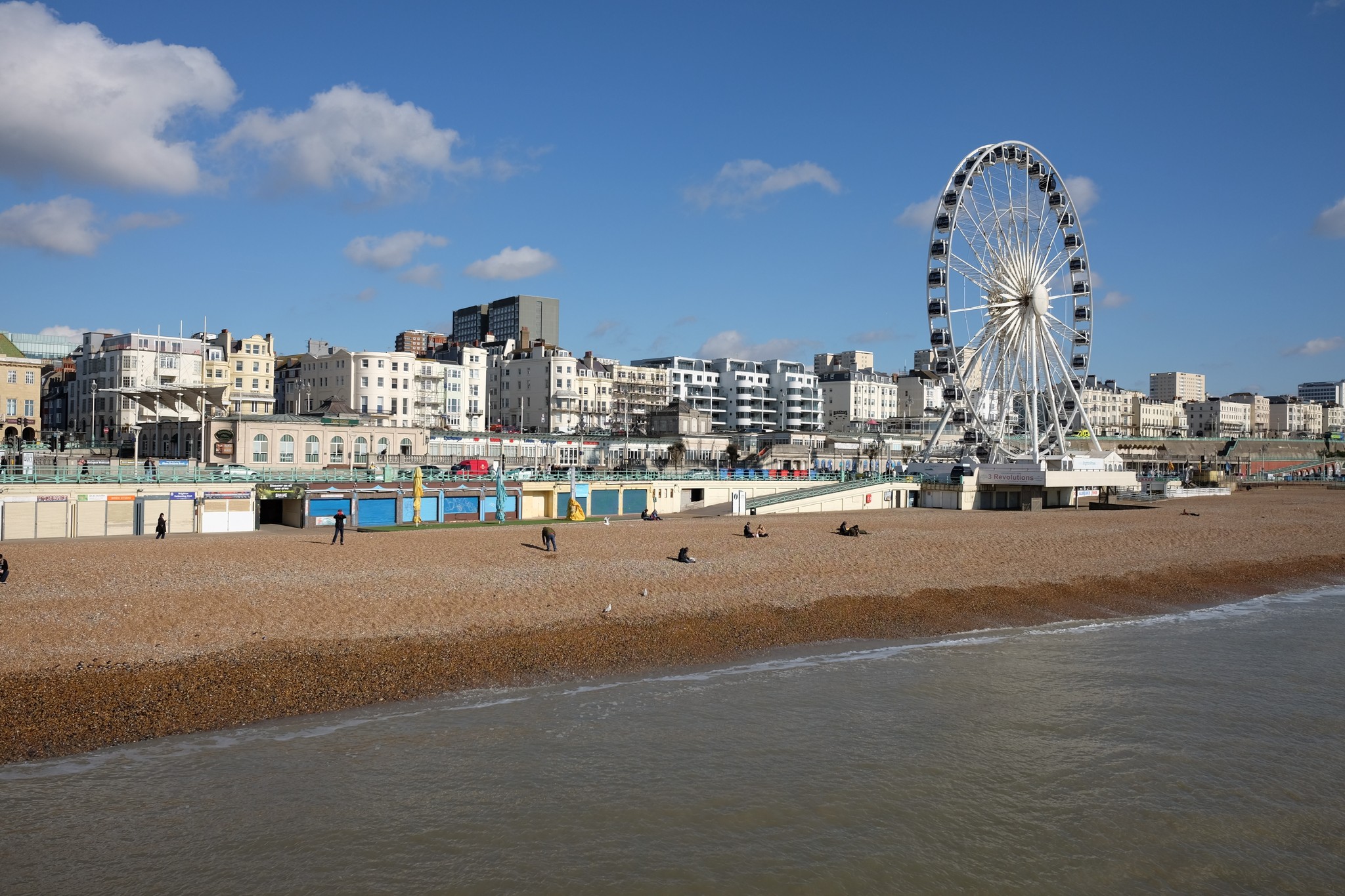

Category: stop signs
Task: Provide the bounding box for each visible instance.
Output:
[103,427,109,433]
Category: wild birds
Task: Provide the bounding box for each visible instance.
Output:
[603,516,610,525]
[643,588,647,596]
[602,603,611,613]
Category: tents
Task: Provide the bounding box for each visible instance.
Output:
[864,419,880,425]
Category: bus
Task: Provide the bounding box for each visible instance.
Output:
[1322,431,1344,440]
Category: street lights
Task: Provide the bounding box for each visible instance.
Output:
[872,431,885,481]
[294,375,311,416]
[905,395,914,417]
[470,386,475,432]
[1277,418,1280,435]
[89,379,98,448]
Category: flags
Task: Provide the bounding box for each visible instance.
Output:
[1184,459,1212,472]
[1167,460,1174,471]
[1226,460,1231,471]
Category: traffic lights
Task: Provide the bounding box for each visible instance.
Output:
[8,437,14,448]
[54,462,57,465]
[46,435,67,452]
[24,418,28,427]
[18,438,25,451]
[17,418,22,425]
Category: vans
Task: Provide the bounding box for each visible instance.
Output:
[443,460,489,480]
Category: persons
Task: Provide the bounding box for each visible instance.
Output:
[886,468,896,481]
[156,513,166,539]
[536,464,620,482]
[0,554,9,582]
[542,526,557,551]
[451,464,459,482]
[140,457,156,483]
[743,522,768,537]
[80,460,89,479]
[678,547,691,563]
[369,449,387,474]
[840,521,859,537]
[330,510,346,545]
[641,509,663,520]
[834,468,849,476]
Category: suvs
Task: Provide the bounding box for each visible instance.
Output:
[684,468,711,480]
[505,466,541,483]
[212,464,260,480]
[1230,473,1246,479]
[558,466,583,481]
[401,465,442,480]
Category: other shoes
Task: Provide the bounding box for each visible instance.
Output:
[545,549,550,551]
[554,549,557,551]
[0,581,7,585]
[331,542,334,545]
[341,543,344,545]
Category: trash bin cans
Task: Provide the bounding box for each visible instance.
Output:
[730,468,745,480]
[788,470,800,481]
[367,469,375,482]
[1338,477,1344,482]
[777,469,788,481]
[906,476,913,483]
[713,468,728,480]
[798,470,808,481]
[754,469,770,481]
[750,508,756,515]
[745,469,755,481]
[1284,476,1292,481]
[807,470,817,481]
[768,469,777,481]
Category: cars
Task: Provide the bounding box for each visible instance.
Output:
[1276,472,1345,482]
[484,424,635,436]
[1141,473,1182,479]
[710,427,823,433]
[808,468,881,479]
[1070,429,1178,438]
